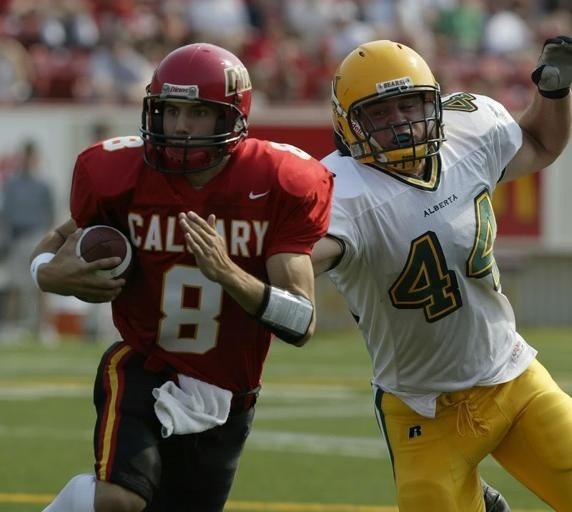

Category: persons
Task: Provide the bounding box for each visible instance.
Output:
[0,1,572,111]
[25,40,333,512]
[1,137,58,347]
[305,36,572,510]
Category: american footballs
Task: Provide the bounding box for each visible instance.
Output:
[75,224,136,284]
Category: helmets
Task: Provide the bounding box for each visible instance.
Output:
[332,40,447,174]
[139,42,252,174]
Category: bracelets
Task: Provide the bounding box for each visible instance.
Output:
[29,249,56,289]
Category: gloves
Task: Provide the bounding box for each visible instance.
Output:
[530,34,572,100]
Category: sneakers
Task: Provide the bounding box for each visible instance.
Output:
[480,476,510,512]
